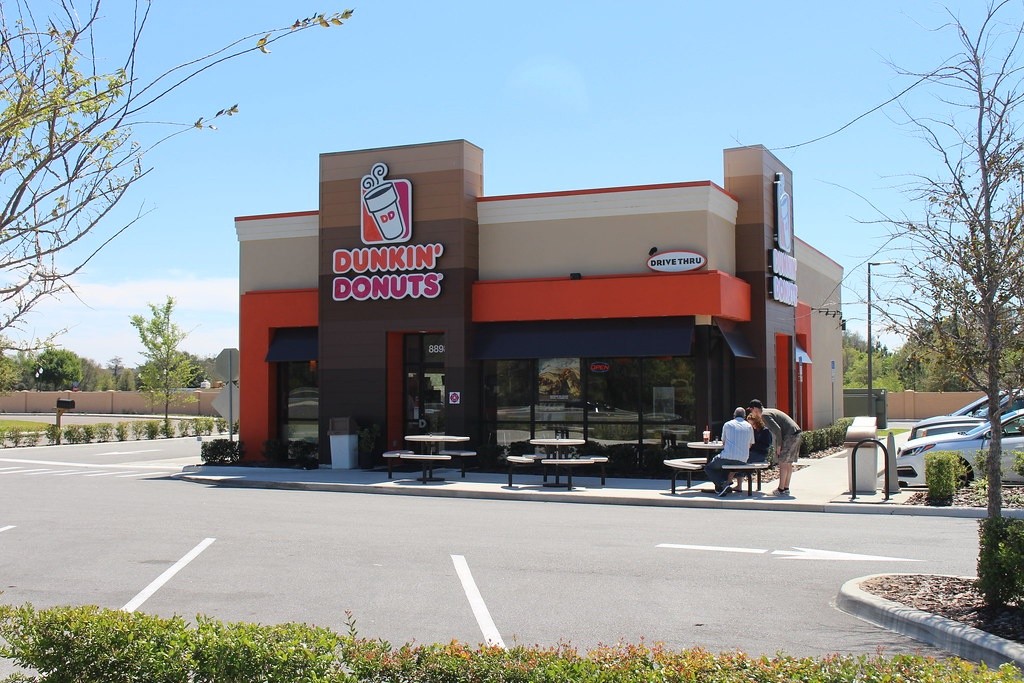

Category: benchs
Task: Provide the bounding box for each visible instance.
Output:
[672,458,708,487]
[400,454,451,484]
[722,464,768,496]
[752,462,770,491]
[568,455,609,486]
[383,450,413,479]
[440,449,477,478]
[523,455,567,482]
[663,460,702,495]
[507,456,534,487]
[541,458,594,490]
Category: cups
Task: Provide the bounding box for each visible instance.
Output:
[703,431,710,444]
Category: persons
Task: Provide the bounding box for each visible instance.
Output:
[747,399,802,496]
[728,413,772,492]
[702,407,756,497]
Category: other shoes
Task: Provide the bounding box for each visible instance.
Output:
[729,482,742,492]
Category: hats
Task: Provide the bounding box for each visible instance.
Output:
[746,399,763,410]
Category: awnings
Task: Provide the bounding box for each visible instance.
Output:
[261,328,322,363]
[465,317,697,360]
[711,316,759,358]
[794,340,812,364]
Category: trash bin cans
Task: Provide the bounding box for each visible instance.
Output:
[326,417,360,470]
[844,416,879,494]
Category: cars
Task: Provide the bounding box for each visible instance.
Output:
[948,389,1024,417]
[907,393,1024,442]
[898,408,1024,487]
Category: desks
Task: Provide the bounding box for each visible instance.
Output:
[687,441,724,493]
[530,438,585,487]
[542,425,579,439]
[404,435,470,481]
[647,429,689,445]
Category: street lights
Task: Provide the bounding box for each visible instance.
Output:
[866,261,901,417]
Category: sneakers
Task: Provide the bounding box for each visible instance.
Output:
[714,482,731,497]
[767,487,790,496]
